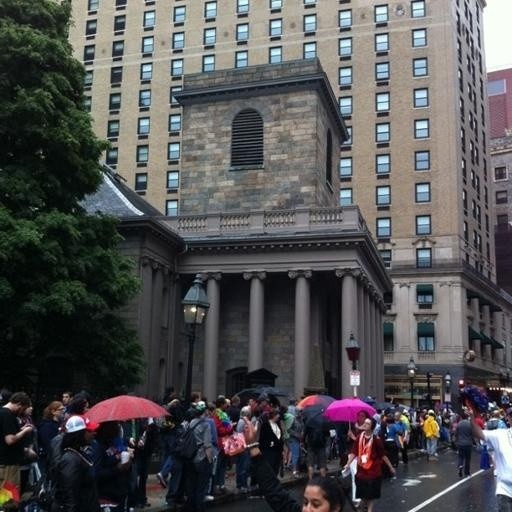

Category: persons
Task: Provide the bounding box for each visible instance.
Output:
[0,390,511,512]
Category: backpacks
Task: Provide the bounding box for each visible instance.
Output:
[177,421,203,459]
[33,453,59,509]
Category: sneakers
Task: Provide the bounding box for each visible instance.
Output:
[459,464,463,469]
[167,485,232,508]
[158,473,168,488]
[464,471,470,475]
[293,470,304,479]
[137,500,150,508]
[239,484,259,493]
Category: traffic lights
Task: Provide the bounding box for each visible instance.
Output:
[457,378,465,388]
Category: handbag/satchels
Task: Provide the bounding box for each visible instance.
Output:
[224,432,246,456]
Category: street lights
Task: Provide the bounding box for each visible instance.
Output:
[406,355,418,410]
[179,270,211,415]
[345,332,361,399]
[444,370,453,413]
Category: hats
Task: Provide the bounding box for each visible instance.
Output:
[428,410,434,414]
[65,415,101,434]
